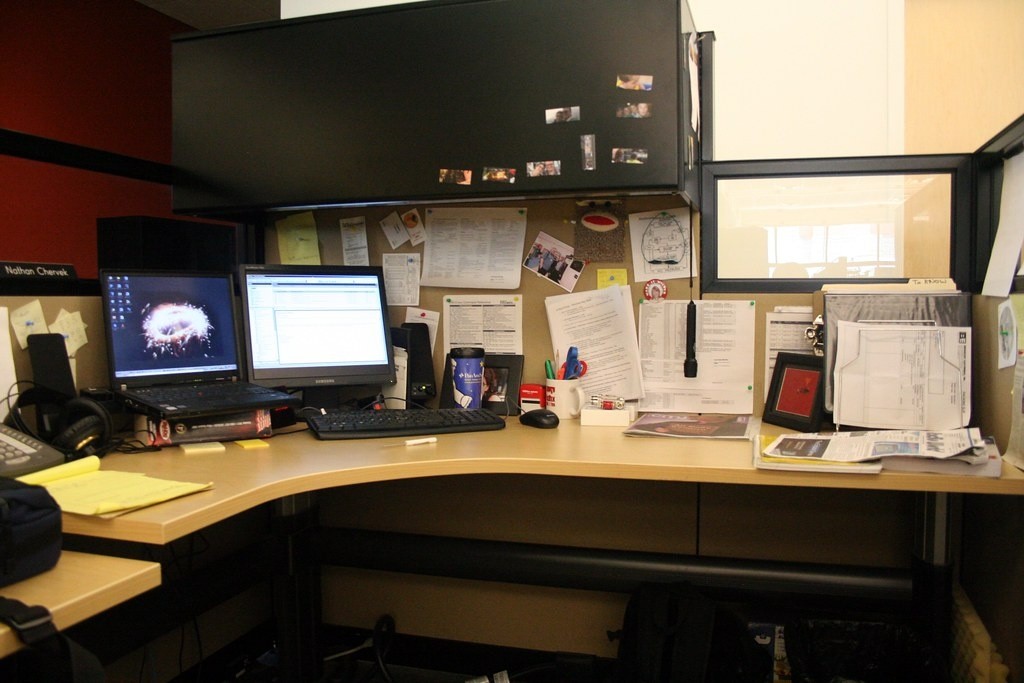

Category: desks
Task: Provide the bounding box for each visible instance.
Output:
[0,550,162,658]
[28,411,1024,683]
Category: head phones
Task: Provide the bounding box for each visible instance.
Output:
[7,380,116,464]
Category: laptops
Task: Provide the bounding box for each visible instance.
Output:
[98,270,301,418]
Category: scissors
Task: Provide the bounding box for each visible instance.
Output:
[566,347,581,380]
[563,361,587,379]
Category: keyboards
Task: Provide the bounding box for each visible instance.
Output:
[306,410,505,440]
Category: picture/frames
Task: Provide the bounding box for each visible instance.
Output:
[763,352,828,433]
[439,353,523,416]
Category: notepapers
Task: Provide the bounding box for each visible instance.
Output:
[180,441,225,453]
[234,439,269,449]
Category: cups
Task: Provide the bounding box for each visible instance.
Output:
[545,378,584,421]
[450,347,485,410]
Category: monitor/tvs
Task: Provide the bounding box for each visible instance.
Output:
[240,265,397,409]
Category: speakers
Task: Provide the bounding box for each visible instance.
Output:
[401,322,438,409]
[28,331,77,402]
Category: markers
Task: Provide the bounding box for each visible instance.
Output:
[545,360,555,379]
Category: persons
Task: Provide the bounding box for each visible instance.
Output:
[532,244,566,282]
[616,103,652,118]
[614,149,646,163]
[483,370,501,401]
[553,107,576,123]
[527,161,560,176]
[616,74,652,91]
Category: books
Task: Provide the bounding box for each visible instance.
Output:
[752,435,1002,477]
[622,413,753,441]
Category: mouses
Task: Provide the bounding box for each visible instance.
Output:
[519,409,559,429]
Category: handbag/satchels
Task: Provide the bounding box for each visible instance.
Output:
[0,476,62,588]
